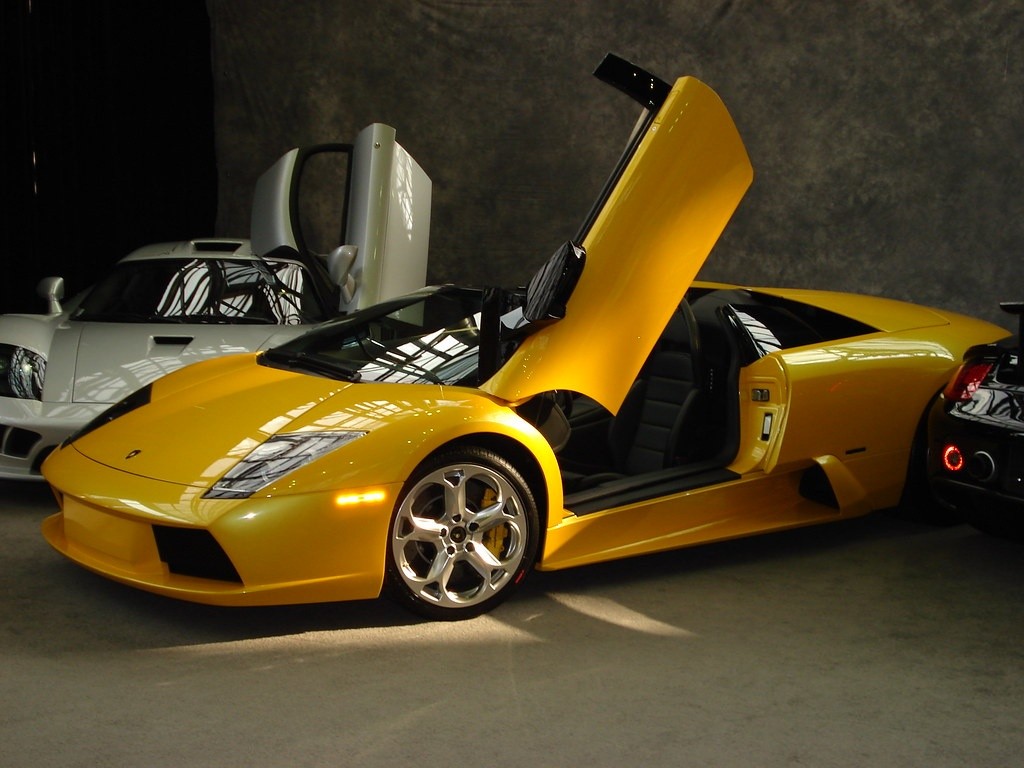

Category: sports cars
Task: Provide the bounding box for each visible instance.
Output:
[39,49,1014,621]
[0,122,433,484]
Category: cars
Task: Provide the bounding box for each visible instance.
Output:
[920,301,1024,534]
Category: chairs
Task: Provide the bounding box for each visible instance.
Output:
[607,296,706,478]
[567,394,602,426]
